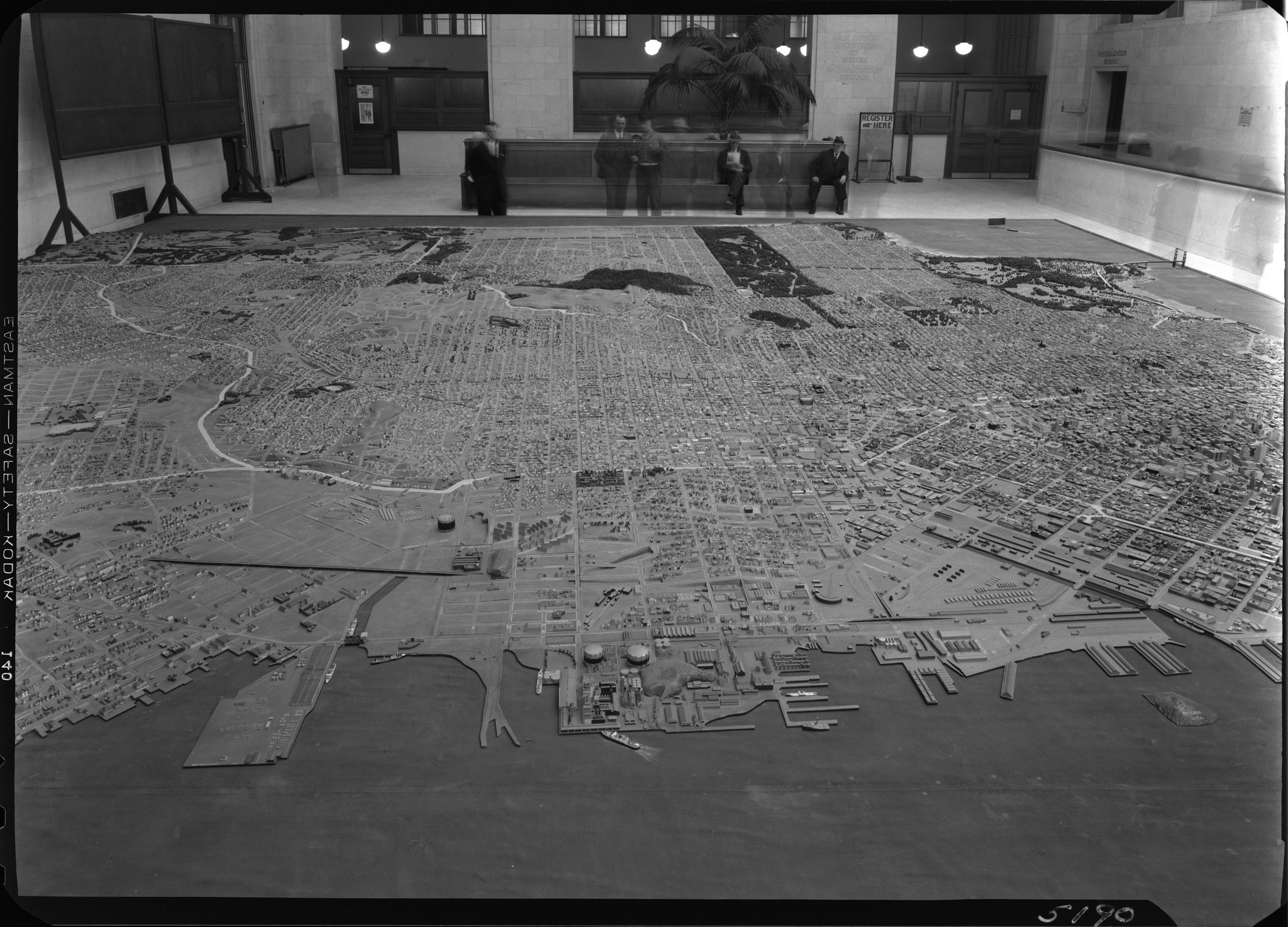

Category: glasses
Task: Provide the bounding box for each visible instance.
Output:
[835,145,843,147]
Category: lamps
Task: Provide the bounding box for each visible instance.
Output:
[800,45,806,56]
[913,14,928,57]
[342,36,349,50]
[776,18,791,56]
[645,14,661,55]
[376,15,390,53]
[955,14,972,55]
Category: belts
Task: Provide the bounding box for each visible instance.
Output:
[639,162,659,166]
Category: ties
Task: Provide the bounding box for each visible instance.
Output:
[618,134,621,140]
[834,154,837,161]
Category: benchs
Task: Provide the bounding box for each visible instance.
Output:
[459,169,850,215]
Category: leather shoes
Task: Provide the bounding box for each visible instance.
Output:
[727,196,734,205]
[836,206,844,215]
[736,206,742,215]
[809,205,816,214]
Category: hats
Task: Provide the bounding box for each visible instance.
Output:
[831,136,848,145]
[728,131,742,142]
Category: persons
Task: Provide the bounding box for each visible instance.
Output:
[632,114,670,216]
[594,114,635,216]
[1225,152,1280,276]
[466,122,507,215]
[756,131,794,217]
[807,136,849,215]
[852,128,889,217]
[1150,141,1202,250]
[717,130,752,216]
